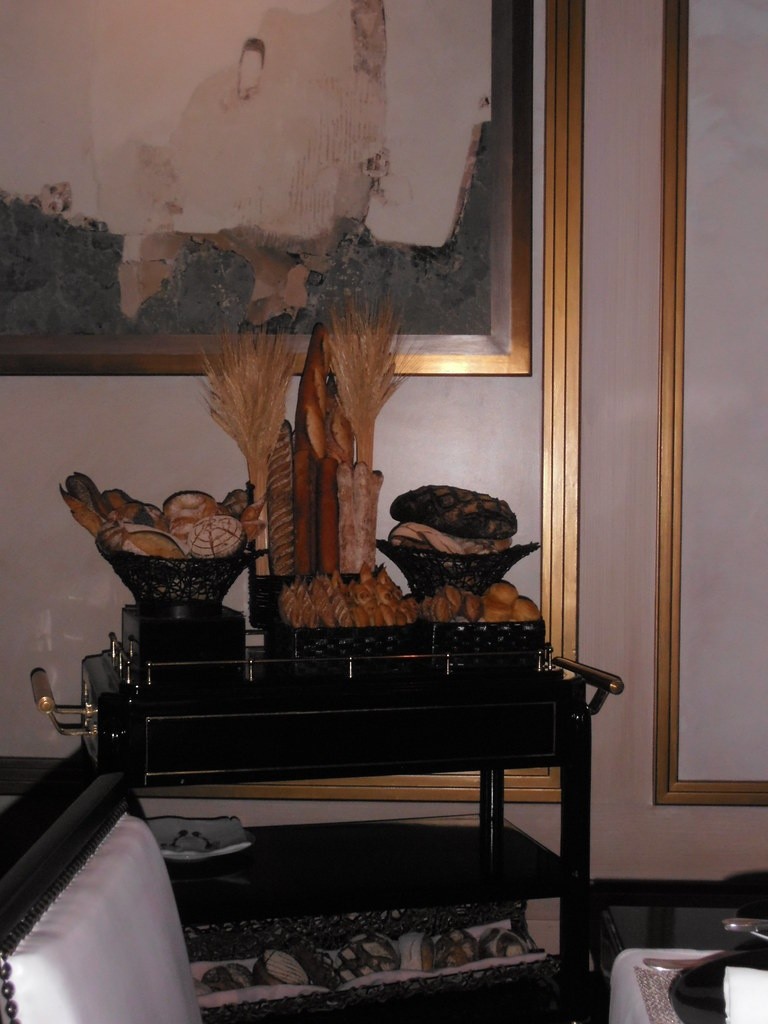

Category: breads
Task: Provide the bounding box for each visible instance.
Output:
[387,485,516,555]
[189,927,526,995]
[423,582,541,623]
[278,562,418,627]
[265,323,384,577]
[58,471,264,559]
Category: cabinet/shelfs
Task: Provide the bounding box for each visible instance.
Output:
[32,629,626,1024]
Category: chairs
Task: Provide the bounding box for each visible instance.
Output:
[1,770,204,1023]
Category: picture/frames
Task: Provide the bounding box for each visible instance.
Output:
[0,1,534,378]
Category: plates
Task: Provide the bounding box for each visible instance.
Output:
[149,817,252,861]
